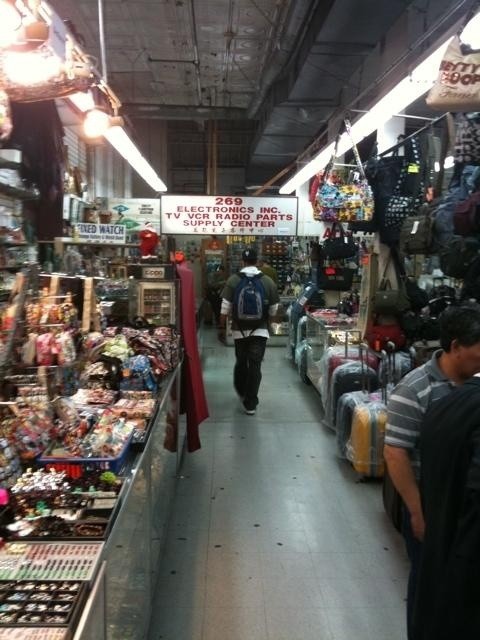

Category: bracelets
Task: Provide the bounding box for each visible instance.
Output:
[76,523,106,537]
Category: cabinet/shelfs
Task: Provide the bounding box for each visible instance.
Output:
[200,233,321,348]
[0,224,184,640]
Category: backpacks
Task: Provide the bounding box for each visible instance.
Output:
[231,268,268,330]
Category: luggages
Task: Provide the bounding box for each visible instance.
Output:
[300,345,313,386]
[335,342,371,463]
[294,315,308,364]
[331,342,378,429]
[348,349,389,486]
[327,329,379,395]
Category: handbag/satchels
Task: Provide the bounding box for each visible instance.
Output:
[317,267,354,290]
[382,466,416,533]
[426,36,478,112]
[348,156,421,232]
[401,113,478,278]
[372,279,411,311]
[314,119,374,221]
[403,279,429,309]
[364,313,410,353]
[0,271,182,392]
[321,223,357,259]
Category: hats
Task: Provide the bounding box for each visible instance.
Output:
[239,248,259,261]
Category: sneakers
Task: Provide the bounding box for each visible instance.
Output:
[240,400,256,415]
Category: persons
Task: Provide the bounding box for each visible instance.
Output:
[406,369,480,640]
[217,247,280,416]
[260,253,280,288]
[204,263,227,330]
[381,298,480,639]
